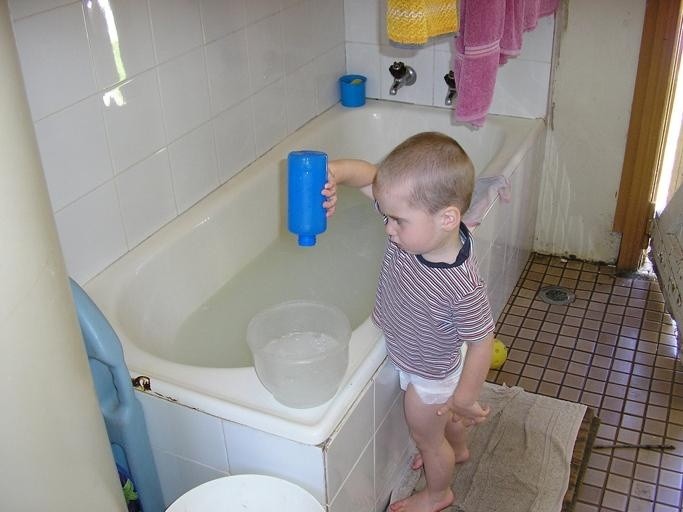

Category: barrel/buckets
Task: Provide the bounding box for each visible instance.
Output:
[245,300,352,408]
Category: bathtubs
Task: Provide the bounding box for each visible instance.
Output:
[84,98,546,446]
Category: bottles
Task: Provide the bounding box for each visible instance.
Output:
[286,149,328,249]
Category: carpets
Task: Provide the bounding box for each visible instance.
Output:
[388,375,599,512]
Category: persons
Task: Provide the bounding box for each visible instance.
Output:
[322,129,495,511]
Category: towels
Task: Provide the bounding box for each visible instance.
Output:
[382,0,559,129]
[462,173,513,230]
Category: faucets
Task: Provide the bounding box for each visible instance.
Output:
[444,69,460,103]
[388,56,417,96]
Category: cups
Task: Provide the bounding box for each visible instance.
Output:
[339,75,367,107]
